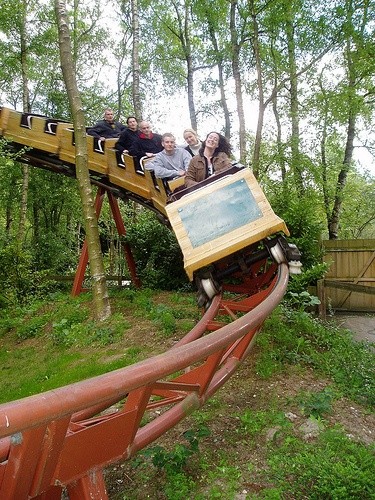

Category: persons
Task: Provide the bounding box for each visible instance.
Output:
[86,108,236,188]
